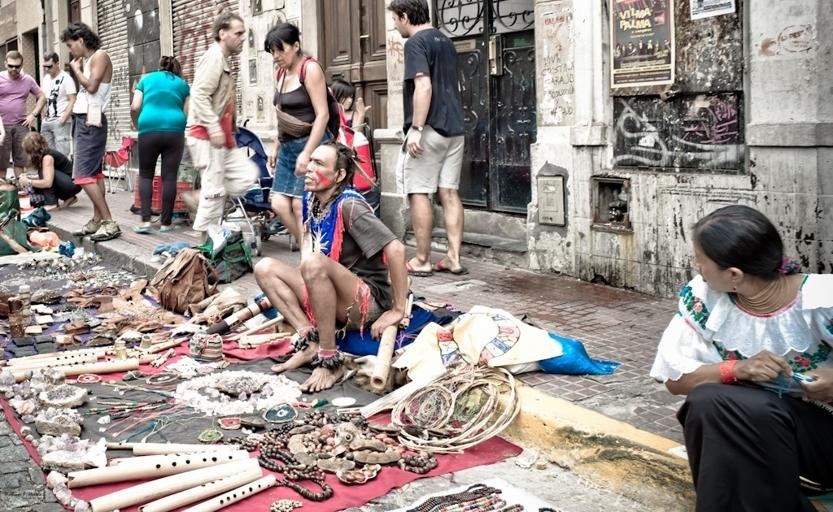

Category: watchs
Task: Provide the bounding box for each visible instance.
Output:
[412,125,424,132]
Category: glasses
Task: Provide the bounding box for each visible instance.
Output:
[8,64,22,69]
[43,65,53,69]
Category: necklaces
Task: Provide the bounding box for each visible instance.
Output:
[738,274,790,314]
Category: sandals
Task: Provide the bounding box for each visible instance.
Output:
[62,196,78,208]
[43,206,60,212]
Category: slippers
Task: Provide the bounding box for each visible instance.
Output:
[432,261,468,275]
[405,261,432,278]
[160,227,177,232]
[134,225,154,234]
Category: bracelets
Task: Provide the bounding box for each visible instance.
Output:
[717,359,738,385]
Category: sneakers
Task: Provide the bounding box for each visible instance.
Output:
[91,220,122,242]
[71,221,102,237]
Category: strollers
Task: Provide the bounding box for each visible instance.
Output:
[193,124,297,257]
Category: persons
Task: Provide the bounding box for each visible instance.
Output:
[183,11,261,246]
[253,139,407,394]
[385,0,467,278]
[1,21,122,242]
[329,78,372,131]
[130,55,191,235]
[650,204,832,512]
[263,22,340,256]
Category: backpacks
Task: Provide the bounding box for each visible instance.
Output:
[159,247,205,315]
[192,227,253,284]
[324,84,340,141]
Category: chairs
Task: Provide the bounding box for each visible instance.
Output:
[98,136,137,194]
